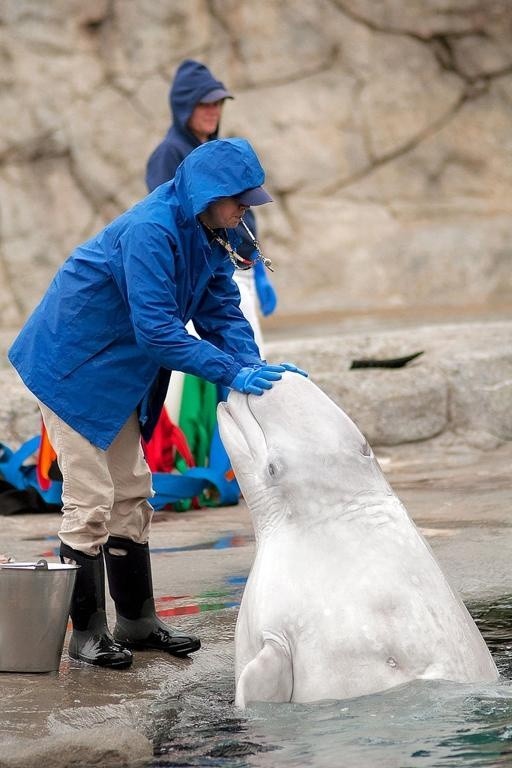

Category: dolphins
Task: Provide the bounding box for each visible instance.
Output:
[212,364,504,713]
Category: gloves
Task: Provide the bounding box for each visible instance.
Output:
[254,270,276,317]
[231,362,309,395]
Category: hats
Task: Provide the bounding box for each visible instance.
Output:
[240,185,273,204]
[200,88,237,104]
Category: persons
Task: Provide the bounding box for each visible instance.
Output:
[144,60,267,430]
[6,136,309,669]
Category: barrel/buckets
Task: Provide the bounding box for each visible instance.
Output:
[0,557,82,673]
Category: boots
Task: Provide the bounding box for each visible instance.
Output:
[102,536,201,657]
[60,542,132,669]
